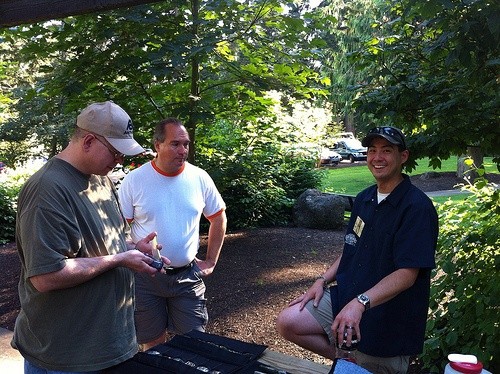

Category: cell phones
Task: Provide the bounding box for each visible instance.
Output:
[141,253,162,270]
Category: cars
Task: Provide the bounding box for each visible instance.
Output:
[330,139,368,163]
[0,162,7,172]
[334,131,354,139]
[319,148,343,166]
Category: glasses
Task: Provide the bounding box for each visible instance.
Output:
[369,127,405,146]
[94,135,126,159]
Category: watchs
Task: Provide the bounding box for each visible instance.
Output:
[356,294,370,311]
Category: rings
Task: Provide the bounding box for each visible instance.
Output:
[346,326,353,329]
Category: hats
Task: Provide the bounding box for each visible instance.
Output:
[362,126,405,146]
[77,100,145,156]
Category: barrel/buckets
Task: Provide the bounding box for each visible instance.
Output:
[444,360,492,374]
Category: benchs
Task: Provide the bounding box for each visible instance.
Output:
[322,192,356,218]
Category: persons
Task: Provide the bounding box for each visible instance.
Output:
[119,119,228,336]
[8,101,172,374]
[276,127,439,374]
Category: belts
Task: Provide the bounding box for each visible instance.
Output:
[165,261,194,275]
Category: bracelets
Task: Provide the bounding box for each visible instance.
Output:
[316,276,327,285]
